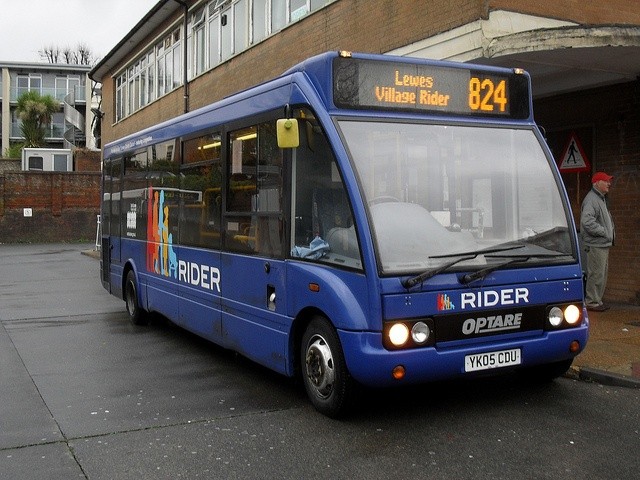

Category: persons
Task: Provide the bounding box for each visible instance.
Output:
[580,172,617,311]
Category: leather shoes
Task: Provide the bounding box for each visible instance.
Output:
[587,305,606,312]
[600,304,609,309]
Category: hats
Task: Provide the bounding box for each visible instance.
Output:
[591,172,614,183]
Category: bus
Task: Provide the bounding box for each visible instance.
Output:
[99,51,589,419]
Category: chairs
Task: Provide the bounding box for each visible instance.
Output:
[317,158,365,238]
